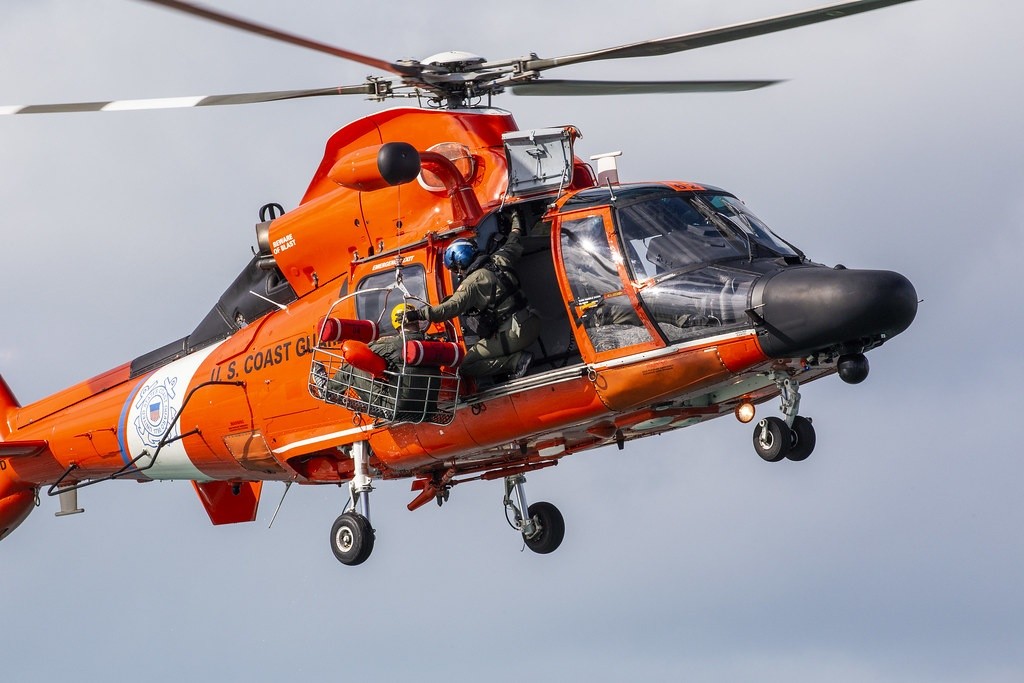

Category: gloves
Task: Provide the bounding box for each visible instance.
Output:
[394,305,426,325]
[501,205,523,231]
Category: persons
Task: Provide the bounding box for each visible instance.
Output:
[312,304,442,418]
[646,204,706,275]
[394,208,538,382]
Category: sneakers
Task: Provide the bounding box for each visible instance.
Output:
[312,360,328,399]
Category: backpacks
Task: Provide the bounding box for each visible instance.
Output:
[464,309,495,337]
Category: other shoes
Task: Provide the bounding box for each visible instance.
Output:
[511,351,533,378]
[476,382,492,393]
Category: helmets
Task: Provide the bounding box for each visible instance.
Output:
[443,237,479,271]
[390,302,419,329]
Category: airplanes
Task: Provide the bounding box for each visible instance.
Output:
[0,1,918,567]
